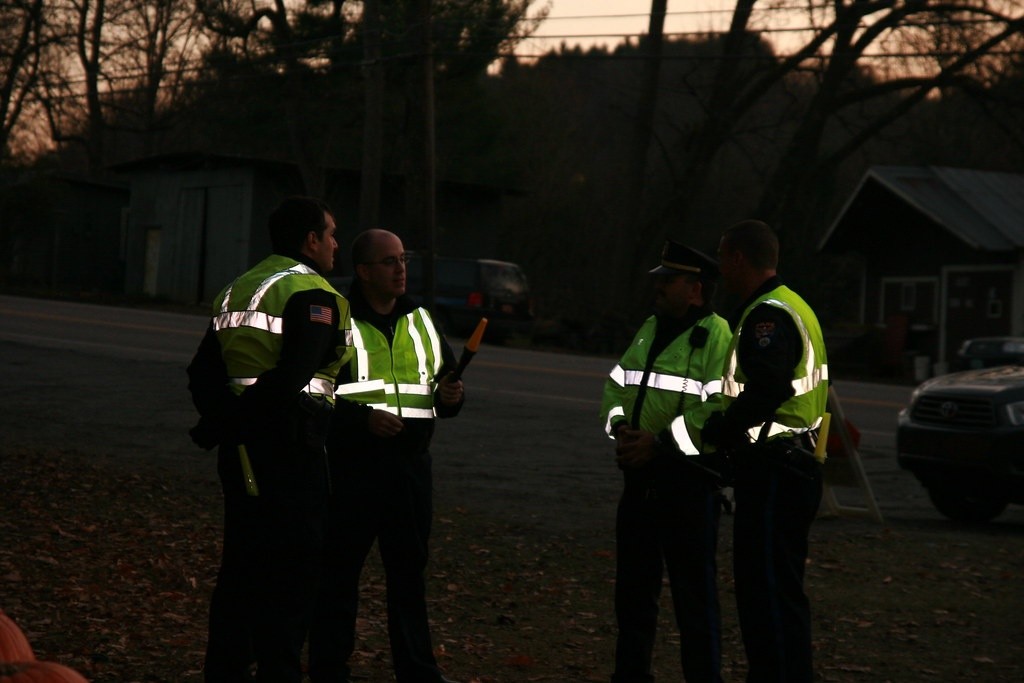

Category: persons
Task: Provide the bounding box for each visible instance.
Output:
[185,193,467,683]
[598,218,829,683]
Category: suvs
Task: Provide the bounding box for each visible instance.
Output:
[398,251,537,349]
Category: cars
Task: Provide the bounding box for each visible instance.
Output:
[895,364,1024,526]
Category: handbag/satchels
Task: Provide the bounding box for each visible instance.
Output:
[266,401,332,482]
[766,440,826,496]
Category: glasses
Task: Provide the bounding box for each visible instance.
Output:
[360,254,410,265]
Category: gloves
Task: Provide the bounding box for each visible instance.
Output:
[188,416,219,451]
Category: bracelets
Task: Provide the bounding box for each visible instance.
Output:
[654,434,664,446]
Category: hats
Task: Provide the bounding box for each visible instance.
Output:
[647,236,721,282]
